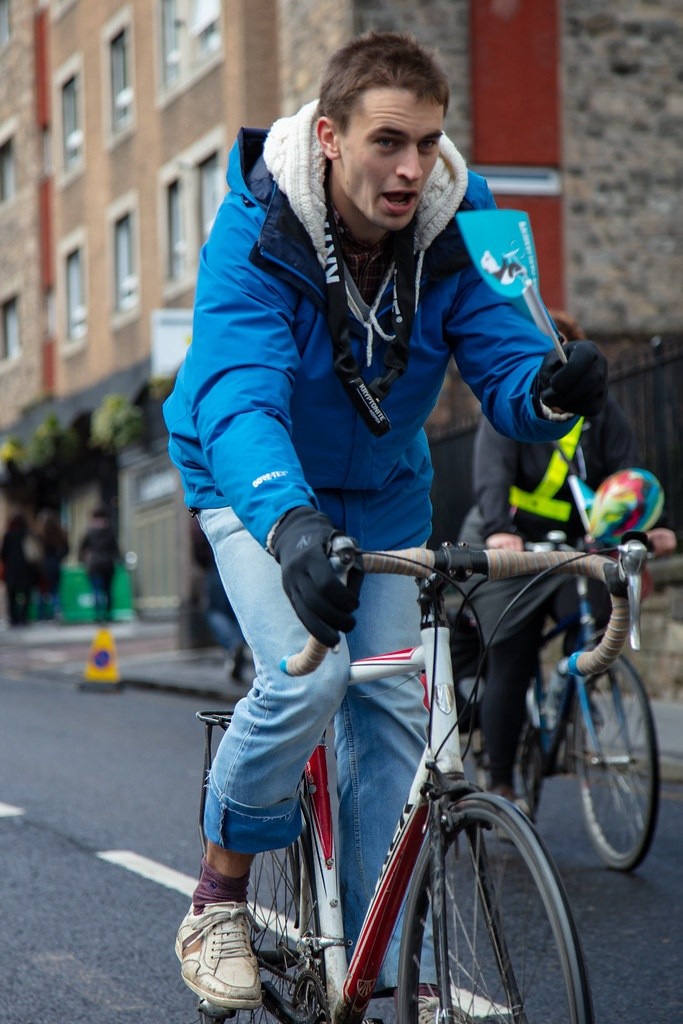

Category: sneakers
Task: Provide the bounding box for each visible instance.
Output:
[418,995,466,1024]
[174,901,262,1010]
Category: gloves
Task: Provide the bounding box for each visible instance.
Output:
[272,504,366,648]
[535,340,608,416]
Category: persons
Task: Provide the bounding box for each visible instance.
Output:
[78,510,134,625]
[156,36,610,1024]
[453,316,677,817]
[187,517,255,682]
[0,513,38,628]
[31,514,68,621]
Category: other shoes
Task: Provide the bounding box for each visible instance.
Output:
[497,786,528,811]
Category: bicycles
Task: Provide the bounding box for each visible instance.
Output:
[463,528,663,873]
[189,531,596,1024]
